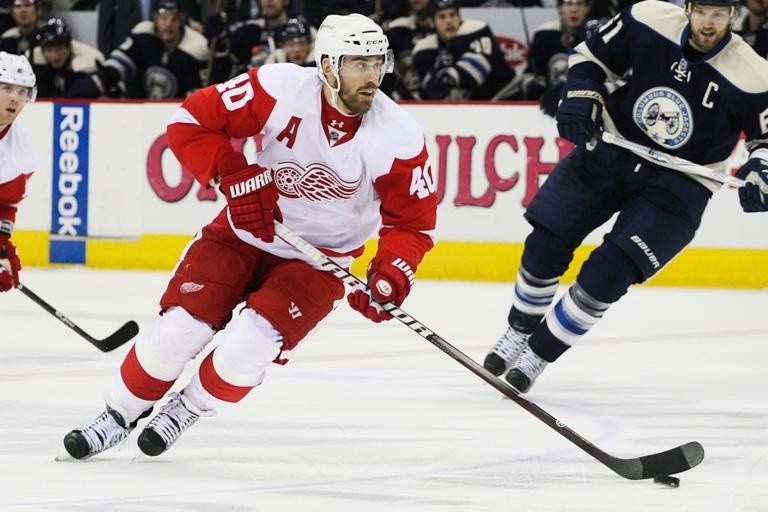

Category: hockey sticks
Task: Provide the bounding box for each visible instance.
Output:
[218,183,705,480]
[12,278,139,353]
[594,129,768,195]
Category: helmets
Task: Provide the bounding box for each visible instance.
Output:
[151,0,186,20]
[0,52,37,102]
[280,16,312,42]
[431,1,460,22]
[557,1,591,8]
[316,14,396,93]
[41,18,71,46]
[683,0,740,23]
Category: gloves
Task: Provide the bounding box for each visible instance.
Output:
[218,163,284,243]
[732,147,768,213]
[1,235,22,296]
[347,251,412,324]
[556,81,609,146]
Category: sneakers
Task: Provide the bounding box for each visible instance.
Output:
[484,324,529,377]
[504,345,550,394]
[137,386,215,456]
[63,408,152,459]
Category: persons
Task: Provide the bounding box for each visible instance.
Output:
[59,12,444,462]
[476,0,768,397]
[1,43,46,300]
